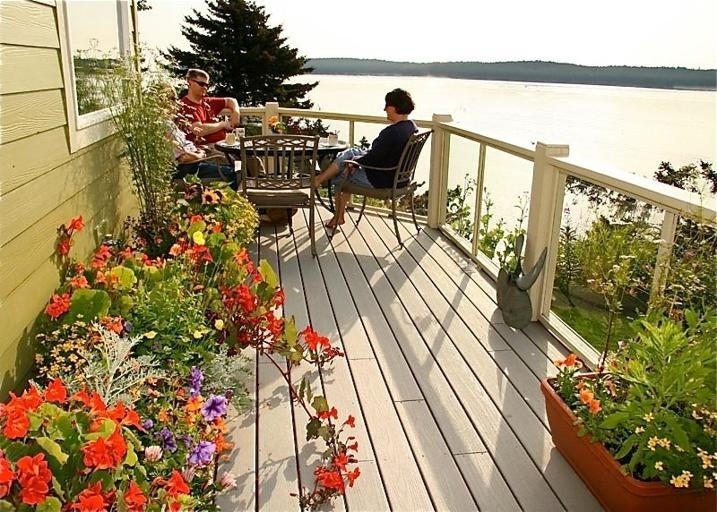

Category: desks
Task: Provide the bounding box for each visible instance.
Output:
[214,132,350,239]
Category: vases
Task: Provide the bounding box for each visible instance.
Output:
[540,370,717,511]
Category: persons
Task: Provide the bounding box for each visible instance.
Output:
[173,67,241,166]
[314,87,420,227]
[164,86,238,192]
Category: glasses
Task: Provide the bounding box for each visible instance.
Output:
[191,80,210,87]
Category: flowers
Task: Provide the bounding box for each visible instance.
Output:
[265,115,286,134]
[553,208,716,488]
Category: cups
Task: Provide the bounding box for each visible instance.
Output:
[328,134,338,146]
[225,133,235,145]
[231,128,245,140]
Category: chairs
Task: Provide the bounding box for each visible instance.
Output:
[166,154,235,189]
[328,129,435,249]
[239,135,317,261]
